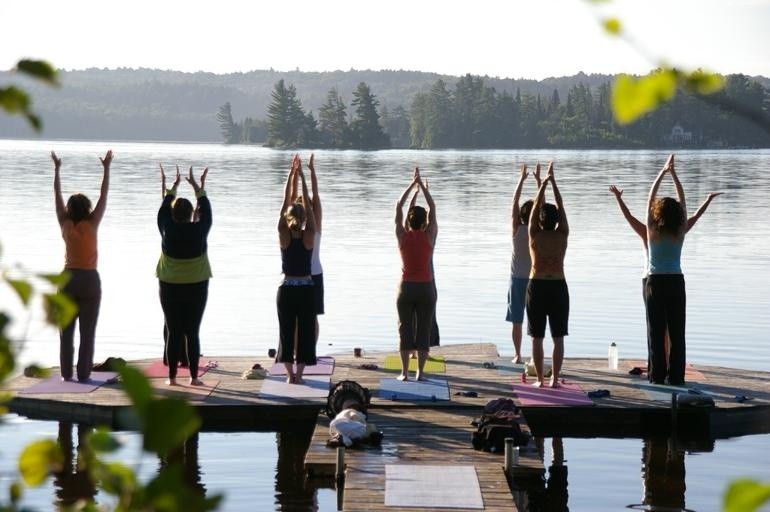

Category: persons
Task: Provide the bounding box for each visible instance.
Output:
[277,154,317,383]
[50,149,115,382]
[404,177,440,360]
[395,168,436,383]
[505,161,546,364]
[608,184,725,380]
[525,162,570,388]
[290,153,323,360]
[645,153,687,385]
[160,164,208,368]
[157,163,212,386]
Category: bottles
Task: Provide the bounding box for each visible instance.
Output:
[608,343,617,369]
[512,446,519,465]
